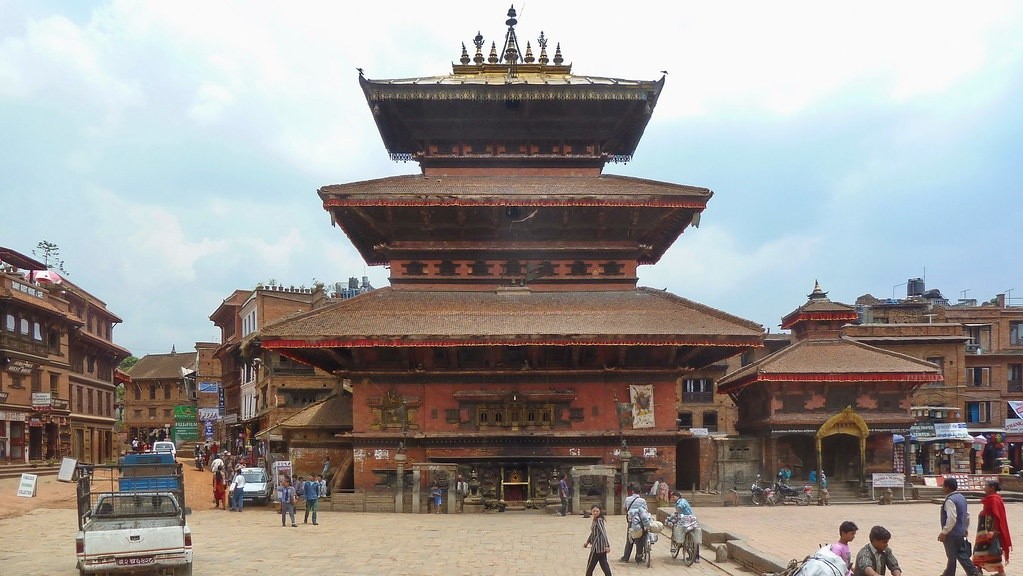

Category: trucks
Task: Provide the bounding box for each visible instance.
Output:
[118,451,185,512]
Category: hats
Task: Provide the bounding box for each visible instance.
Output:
[632,484,641,492]
[309,472,316,477]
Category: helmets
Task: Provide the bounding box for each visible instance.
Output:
[196,445,200,450]
[776,472,783,480]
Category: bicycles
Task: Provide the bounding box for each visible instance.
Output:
[642,530,652,569]
[664,519,698,566]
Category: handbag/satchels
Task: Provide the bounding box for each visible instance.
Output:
[964,538,972,558]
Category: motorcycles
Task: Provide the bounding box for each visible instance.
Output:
[750,484,776,507]
[771,481,813,506]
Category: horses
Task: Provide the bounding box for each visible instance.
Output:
[792,543,853,576]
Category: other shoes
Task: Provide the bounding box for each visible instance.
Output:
[990,571,1006,576]
[617,557,628,563]
[304,519,307,524]
[292,524,298,527]
[556,511,562,516]
[313,522,318,525]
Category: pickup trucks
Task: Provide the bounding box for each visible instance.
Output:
[75,462,193,576]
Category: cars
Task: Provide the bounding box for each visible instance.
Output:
[227,467,274,507]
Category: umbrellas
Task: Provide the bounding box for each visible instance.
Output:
[25,270,62,285]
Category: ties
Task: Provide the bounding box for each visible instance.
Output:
[285,488,290,501]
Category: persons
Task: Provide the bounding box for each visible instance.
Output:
[651,477,669,503]
[132,437,139,451]
[148,496,167,511]
[777,464,791,486]
[281,456,330,527]
[144,442,150,453]
[194,439,246,512]
[830,521,859,576]
[971,480,1013,576]
[821,470,827,488]
[671,492,702,563]
[556,472,569,516]
[854,525,902,576]
[618,482,649,563]
[937,477,983,576]
[583,503,613,576]
[432,484,442,514]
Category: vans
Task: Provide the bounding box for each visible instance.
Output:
[152,441,176,462]
